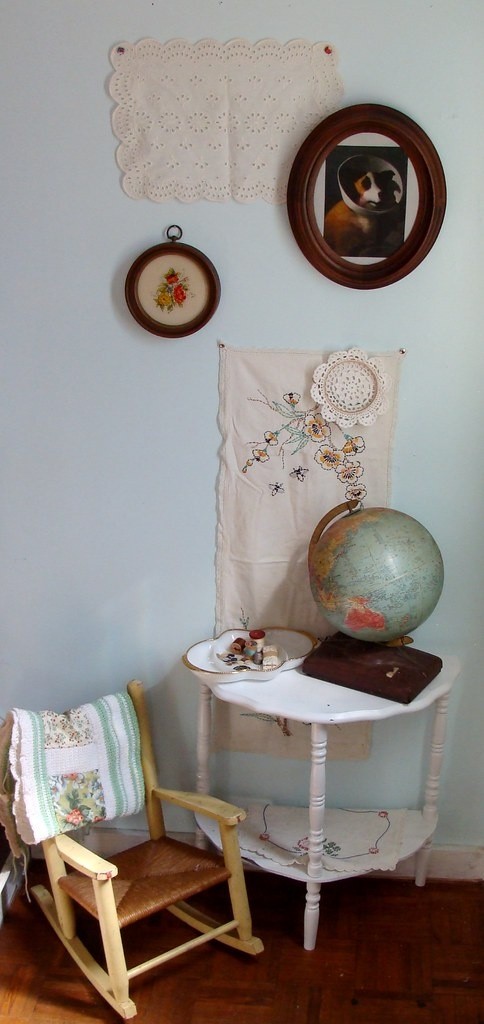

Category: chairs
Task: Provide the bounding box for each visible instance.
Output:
[0,678,265,1020]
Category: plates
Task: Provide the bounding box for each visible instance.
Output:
[182,626,317,683]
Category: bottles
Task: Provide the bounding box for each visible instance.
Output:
[248,629,265,652]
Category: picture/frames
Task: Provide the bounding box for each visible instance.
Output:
[125,242,221,338]
[287,103,448,291]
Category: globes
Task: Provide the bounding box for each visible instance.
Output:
[300,499,444,705]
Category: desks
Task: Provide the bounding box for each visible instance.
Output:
[194,655,460,952]
